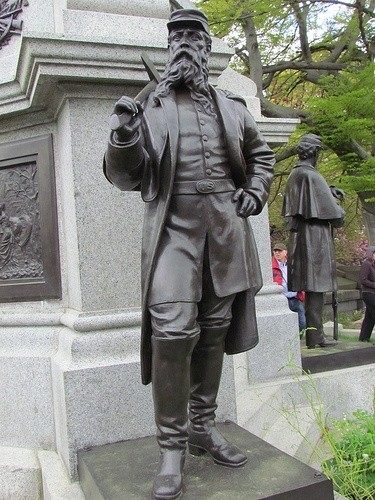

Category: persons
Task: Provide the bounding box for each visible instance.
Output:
[281,133,346,349]
[104,9,276,500]
[359,246,375,343]
[272,242,306,340]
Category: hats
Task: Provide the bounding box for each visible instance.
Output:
[273,242,286,250]
[367,246,375,259]
